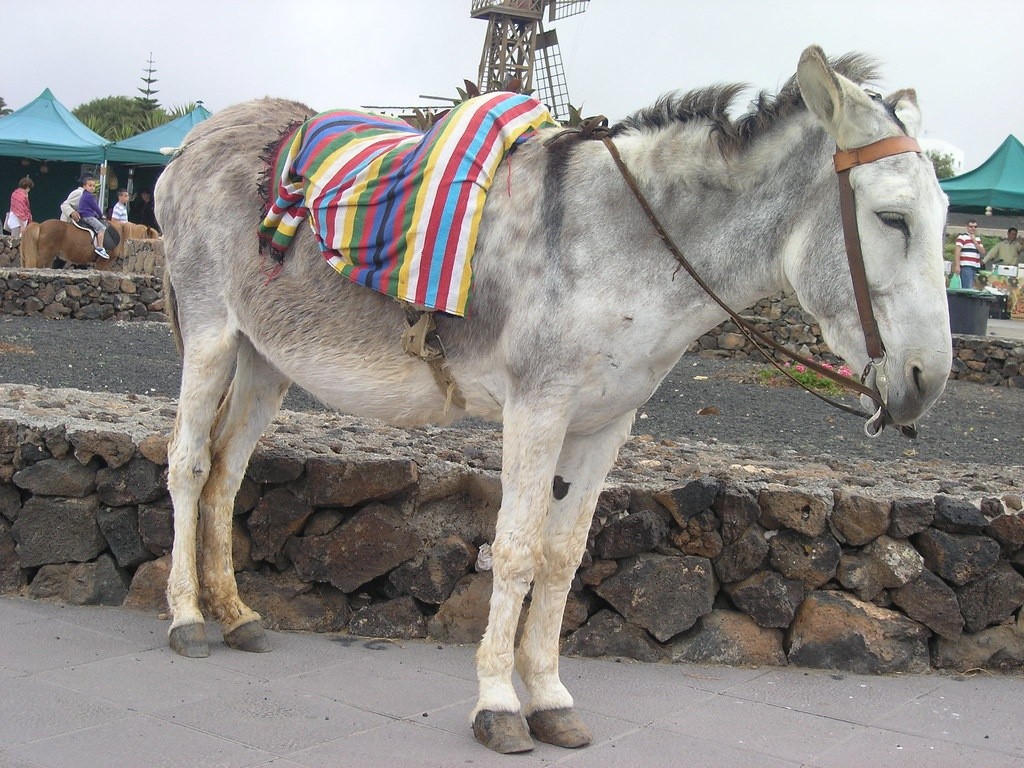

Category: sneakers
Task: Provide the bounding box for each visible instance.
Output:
[95,247,110,260]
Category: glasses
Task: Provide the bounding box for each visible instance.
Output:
[968,225,978,228]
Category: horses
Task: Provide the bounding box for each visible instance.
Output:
[150,46,952,756]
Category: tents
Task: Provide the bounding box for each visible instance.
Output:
[0,88,112,223]
[101,105,214,218]
[935,134,1024,216]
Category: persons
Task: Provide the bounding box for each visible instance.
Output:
[51,172,93,269]
[954,218,986,289]
[7,178,33,238]
[133,188,156,228]
[111,189,128,223]
[79,177,110,258]
[980,226,1024,267]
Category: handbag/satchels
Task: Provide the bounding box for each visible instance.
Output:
[949,272,962,289]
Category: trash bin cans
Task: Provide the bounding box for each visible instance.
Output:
[989,295,1008,319]
[947,289,997,336]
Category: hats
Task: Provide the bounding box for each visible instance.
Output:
[77,171,95,184]
